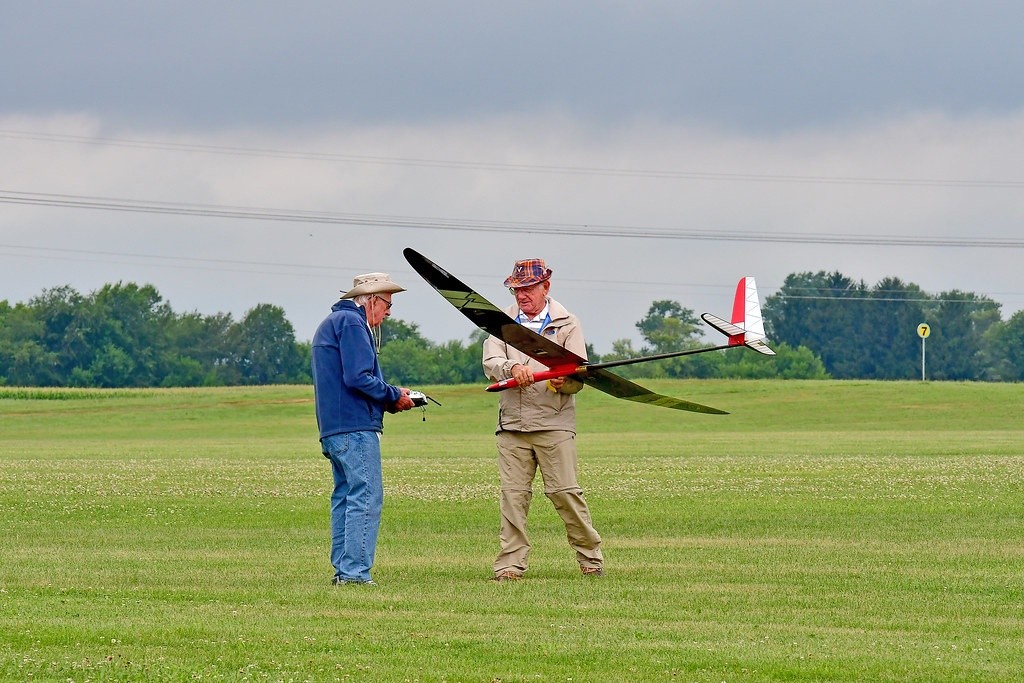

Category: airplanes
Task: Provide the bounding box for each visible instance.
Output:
[403,246,774,415]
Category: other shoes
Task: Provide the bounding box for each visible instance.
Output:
[363,580,377,586]
[587,571,603,576]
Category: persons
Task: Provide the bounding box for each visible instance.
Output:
[482,258,604,581]
[310,273,415,590]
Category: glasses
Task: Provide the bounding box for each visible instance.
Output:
[509,283,540,295]
[368,295,392,309]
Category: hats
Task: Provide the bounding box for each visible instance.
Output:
[504,258,552,287]
[340,273,407,299]
